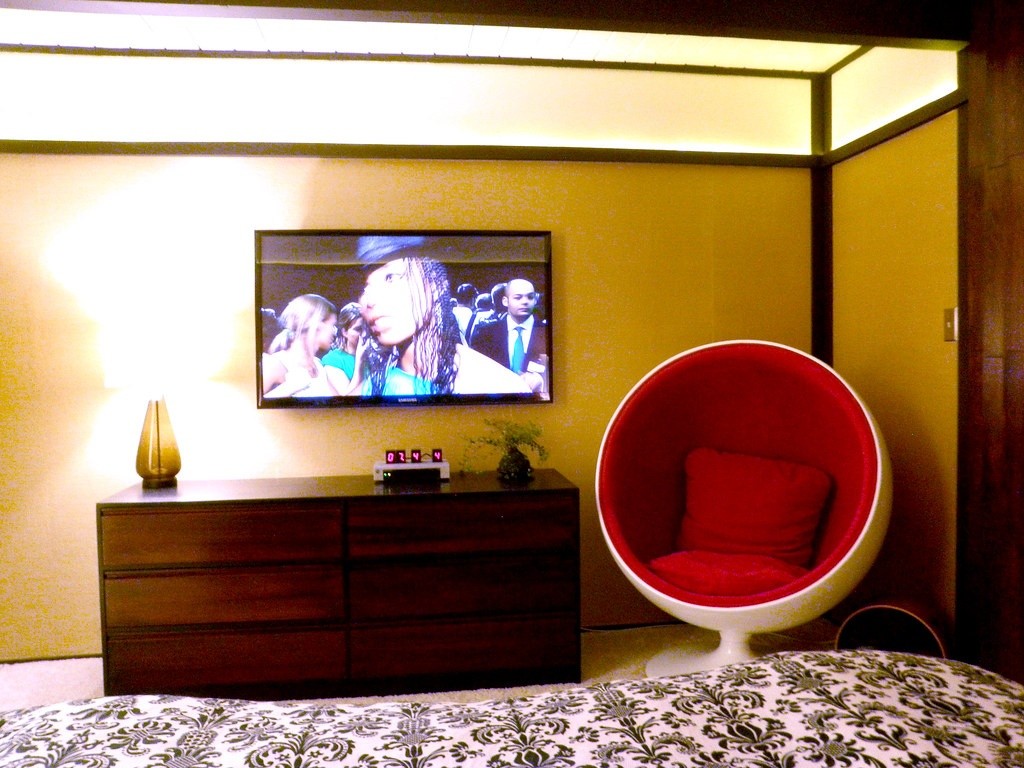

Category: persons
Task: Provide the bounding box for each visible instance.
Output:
[263,236,549,398]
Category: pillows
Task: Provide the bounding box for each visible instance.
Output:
[678,447,830,564]
[650,548,809,596]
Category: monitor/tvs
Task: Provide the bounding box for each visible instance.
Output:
[254,230,553,409]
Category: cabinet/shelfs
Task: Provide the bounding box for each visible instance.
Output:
[96,468,581,701]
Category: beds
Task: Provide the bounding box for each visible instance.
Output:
[0,646,1024,768]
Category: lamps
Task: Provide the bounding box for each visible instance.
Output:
[135,399,181,487]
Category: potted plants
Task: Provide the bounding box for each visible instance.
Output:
[452,413,549,486]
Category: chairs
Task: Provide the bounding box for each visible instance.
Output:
[593,339,893,676]
